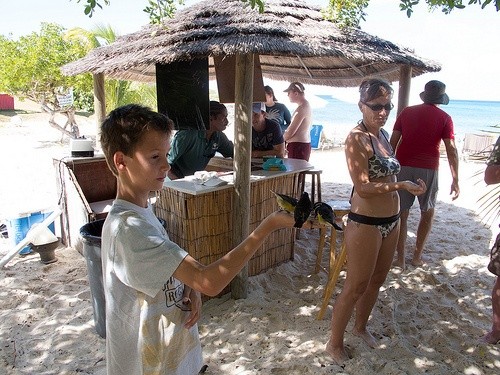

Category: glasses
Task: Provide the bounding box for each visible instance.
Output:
[361,102,394,111]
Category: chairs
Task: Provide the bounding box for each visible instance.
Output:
[310,125,334,151]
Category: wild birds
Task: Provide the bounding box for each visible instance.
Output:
[270,188,343,232]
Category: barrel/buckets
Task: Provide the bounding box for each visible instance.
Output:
[310,125,323,148]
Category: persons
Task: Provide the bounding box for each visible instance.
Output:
[167,101,233,180]
[252,103,285,159]
[389,80,460,270]
[264,85,291,135]
[282,81,312,196]
[484,135,500,343]
[100,103,344,375]
[325,78,428,369]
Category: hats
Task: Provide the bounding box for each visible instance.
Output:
[191,171,228,187]
[252,103,266,113]
[283,81,305,92]
[420,80,449,105]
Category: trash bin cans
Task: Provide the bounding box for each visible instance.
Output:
[80,218,167,338]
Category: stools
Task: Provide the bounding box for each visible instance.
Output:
[316,200,347,321]
[298,170,323,205]
